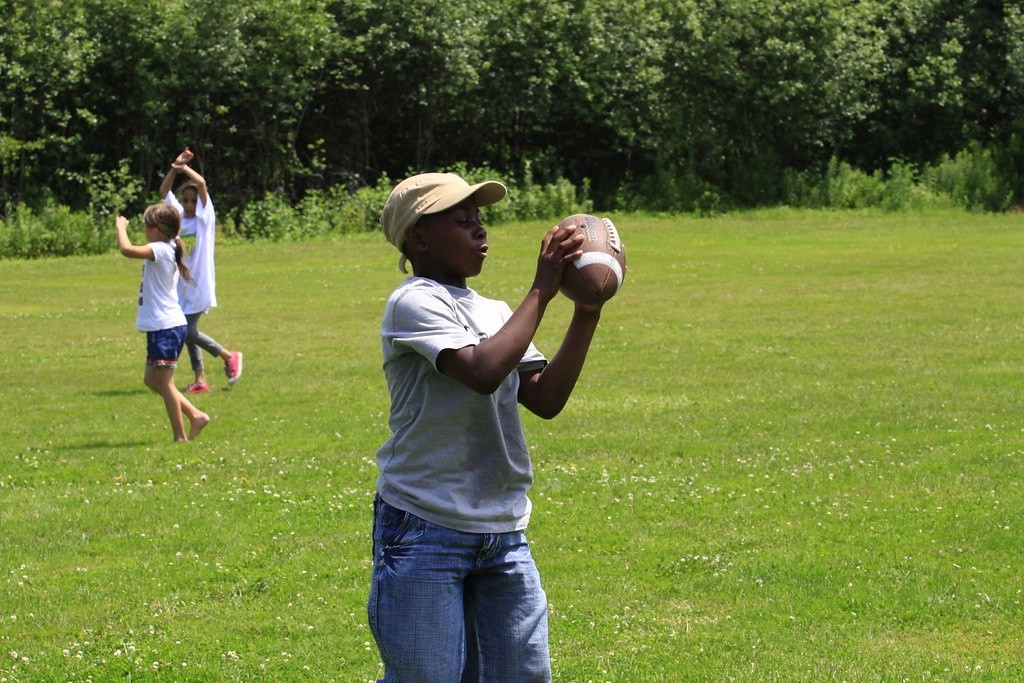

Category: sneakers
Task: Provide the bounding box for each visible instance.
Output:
[182,351,243,391]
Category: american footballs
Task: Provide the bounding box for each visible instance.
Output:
[548,213,627,307]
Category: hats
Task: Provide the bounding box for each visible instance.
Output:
[379,173,507,274]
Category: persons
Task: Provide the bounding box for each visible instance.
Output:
[366,171,606,683]
[159,145,241,395]
[115,202,211,447]
